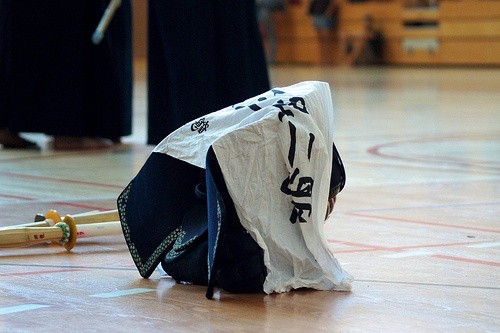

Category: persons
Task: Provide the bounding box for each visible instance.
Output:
[304,0,340,66]
[342,12,385,66]
